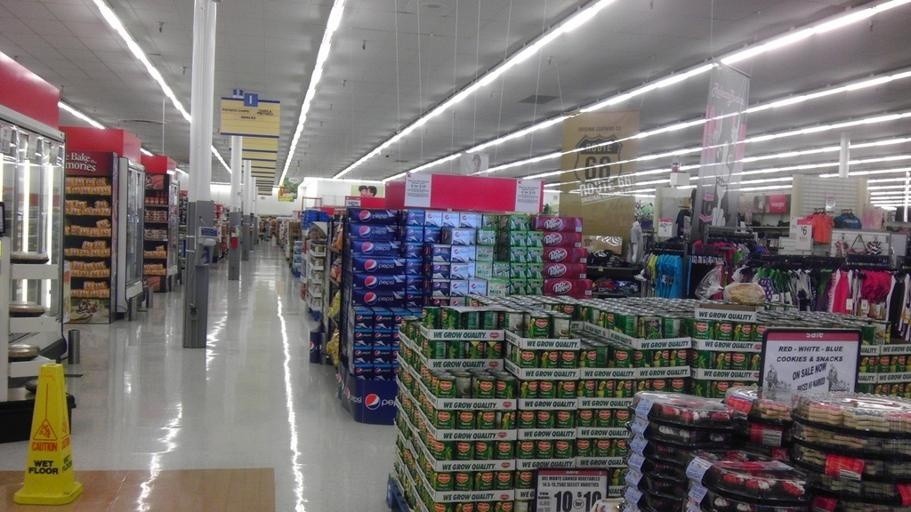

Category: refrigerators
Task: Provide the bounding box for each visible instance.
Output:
[123,161,143,327]
[167,185,182,287]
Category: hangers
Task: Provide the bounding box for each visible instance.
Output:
[638,239,723,262]
[739,253,909,272]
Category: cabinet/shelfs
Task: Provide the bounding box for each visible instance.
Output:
[0,92,67,403]
[141,154,179,295]
[179,190,192,272]
[59,125,145,324]
[275,215,341,365]
